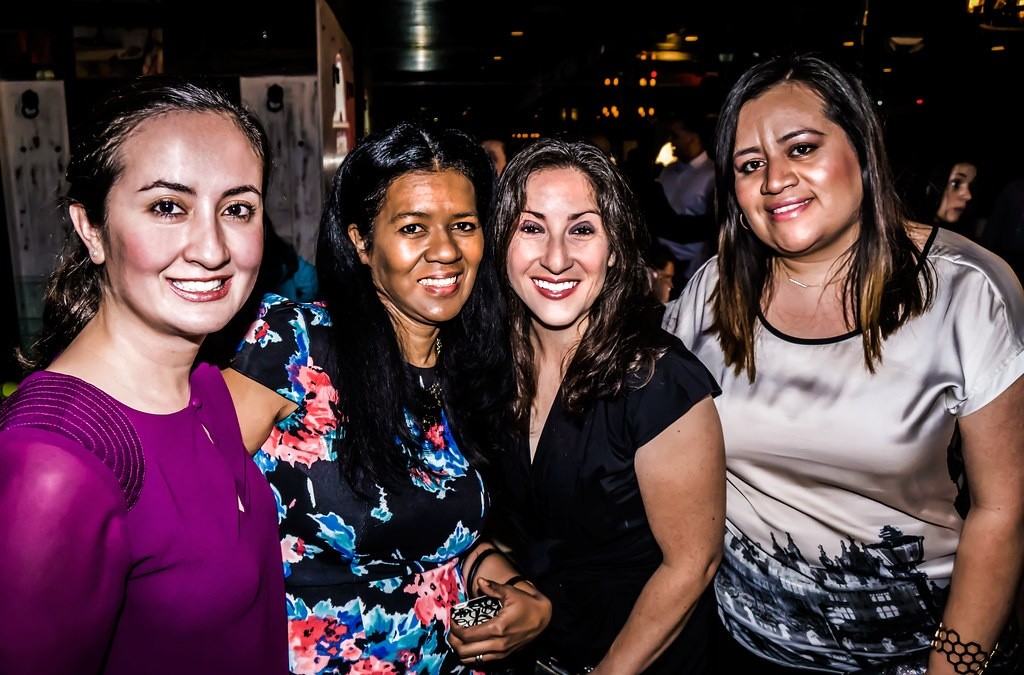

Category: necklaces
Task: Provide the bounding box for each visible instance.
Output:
[782,267,847,288]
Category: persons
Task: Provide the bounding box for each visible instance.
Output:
[0,69,288,675]
[662,56,1024,675]
[656,106,715,285]
[905,138,994,503]
[436,139,727,675]
[221,122,552,675]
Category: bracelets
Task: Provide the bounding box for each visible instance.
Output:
[458,536,494,570]
[503,575,528,589]
[931,622,999,674]
[407,338,448,432]
[467,549,509,599]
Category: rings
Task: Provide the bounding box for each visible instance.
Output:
[476,655,484,666]
